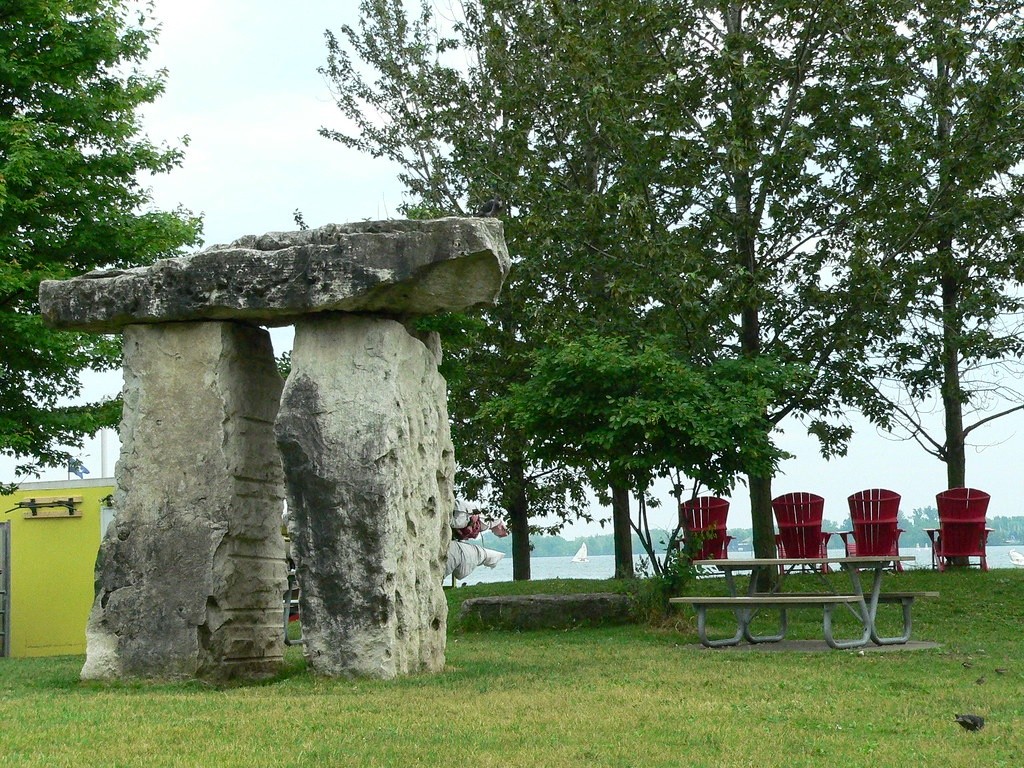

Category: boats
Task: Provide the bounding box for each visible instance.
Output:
[1008,550,1024,567]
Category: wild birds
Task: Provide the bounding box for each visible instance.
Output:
[995,669,1008,673]
[954,714,984,732]
[961,662,972,668]
[975,677,987,685]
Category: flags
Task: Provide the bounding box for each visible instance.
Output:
[69,456,89,480]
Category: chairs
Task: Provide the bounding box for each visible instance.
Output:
[771,492,834,574]
[922,487,996,573]
[835,488,907,574]
[676,496,737,576]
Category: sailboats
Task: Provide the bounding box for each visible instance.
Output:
[570,541,590,563]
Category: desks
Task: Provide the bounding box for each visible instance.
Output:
[693,556,916,647]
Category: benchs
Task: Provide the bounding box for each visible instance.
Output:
[753,591,940,643]
[669,596,864,649]
